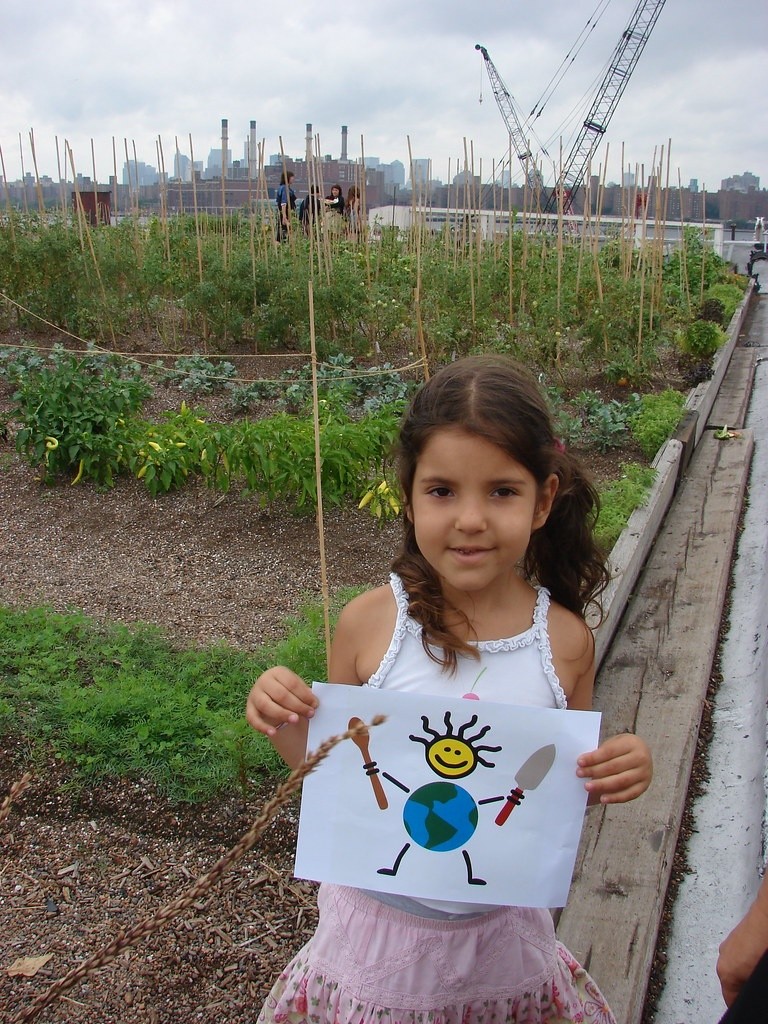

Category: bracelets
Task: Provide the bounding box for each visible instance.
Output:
[266,721,289,739]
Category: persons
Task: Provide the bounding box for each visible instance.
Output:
[300,186,320,235]
[245,357,651,1024]
[716,865,768,1024]
[277,171,296,243]
[345,186,361,243]
[325,186,344,218]
